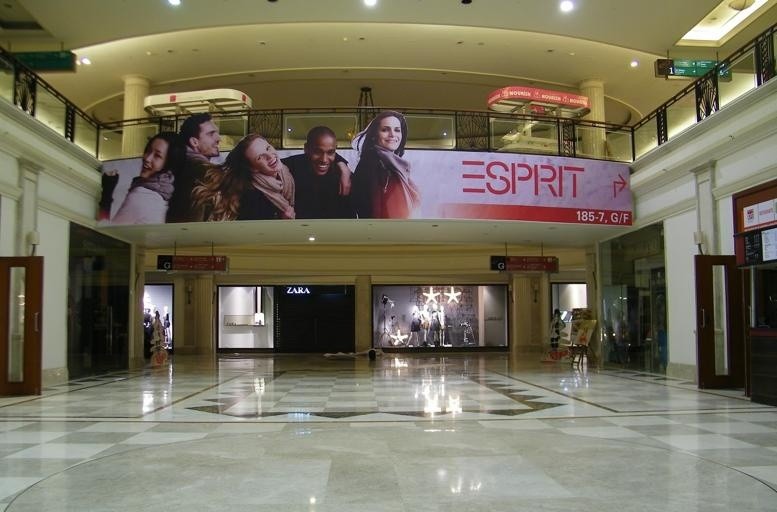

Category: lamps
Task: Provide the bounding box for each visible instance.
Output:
[27,229,40,255]
[694,229,705,254]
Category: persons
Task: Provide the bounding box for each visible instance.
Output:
[142,310,170,351]
[99,130,187,227]
[166,112,221,222]
[380,301,448,348]
[351,109,420,220]
[288,125,355,219]
[549,307,564,346]
[189,134,353,222]
